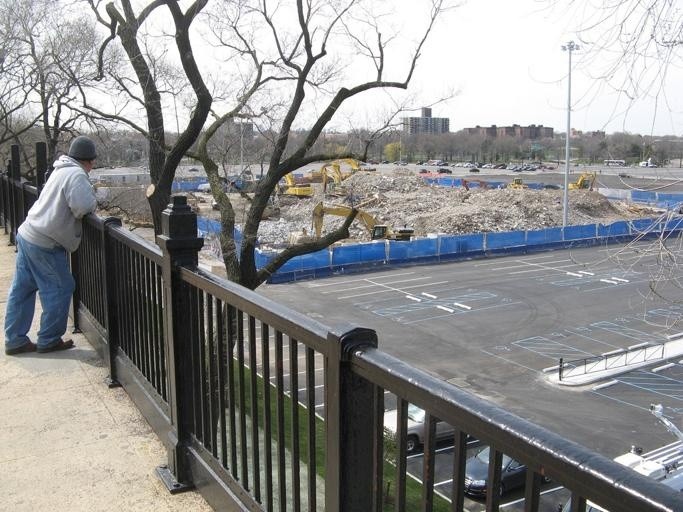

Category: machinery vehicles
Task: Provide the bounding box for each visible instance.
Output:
[506,177,528,189]
[460,177,501,193]
[567,172,595,192]
[272,159,376,197]
[289,200,414,246]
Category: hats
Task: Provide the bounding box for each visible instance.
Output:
[68,136,97,160]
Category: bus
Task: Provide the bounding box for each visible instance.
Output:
[603,159,625,166]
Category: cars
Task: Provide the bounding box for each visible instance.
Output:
[382,398,473,456]
[465,446,553,500]
[639,162,656,168]
[380,158,560,174]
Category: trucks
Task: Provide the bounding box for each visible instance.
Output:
[555,437,682,512]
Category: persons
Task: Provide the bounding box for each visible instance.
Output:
[4,137,98,355]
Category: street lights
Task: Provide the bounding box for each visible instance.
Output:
[557,39,580,229]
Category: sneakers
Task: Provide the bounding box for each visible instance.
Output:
[36,338,72,352]
[6,343,37,354]
